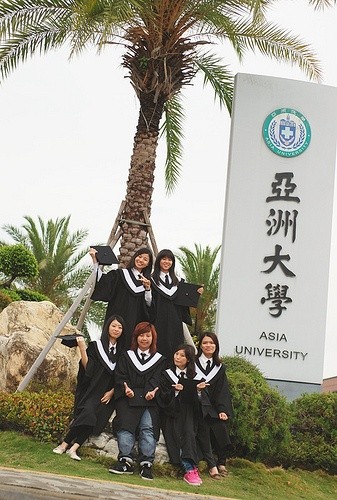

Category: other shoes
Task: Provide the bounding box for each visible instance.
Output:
[66,450,81,461]
[53,445,65,454]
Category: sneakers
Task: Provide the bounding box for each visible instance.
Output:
[184,470,200,486]
[139,461,153,480]
[192,467,202,484]
[109,457,133,475]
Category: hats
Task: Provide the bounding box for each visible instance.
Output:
[55,334,89,348]
[128,387,154,406]
[173,283,204,332]
[90,246,120,283]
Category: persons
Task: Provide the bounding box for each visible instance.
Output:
[53,245,233,486]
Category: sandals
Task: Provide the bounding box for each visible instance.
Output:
[209,470,221,480]
[218,465,227,477]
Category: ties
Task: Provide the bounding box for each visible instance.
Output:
[109,347,114,355]
[141,352,147,360]
[138,274,141,282]
[180,372,185,379]
[165,275,170,284]
[205,360,211,372]
